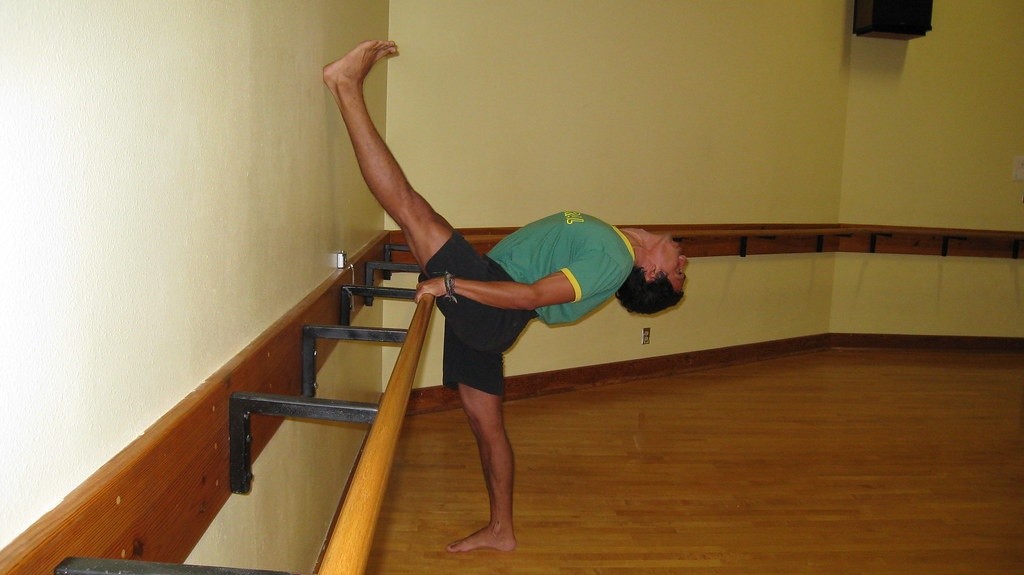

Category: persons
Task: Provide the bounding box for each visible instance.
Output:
[324,41,688,551]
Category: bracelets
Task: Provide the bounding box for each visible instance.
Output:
[443,273,458,304]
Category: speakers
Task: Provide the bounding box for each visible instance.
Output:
[853,0,934,39]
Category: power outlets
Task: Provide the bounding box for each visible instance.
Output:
[642,328,650,344]
